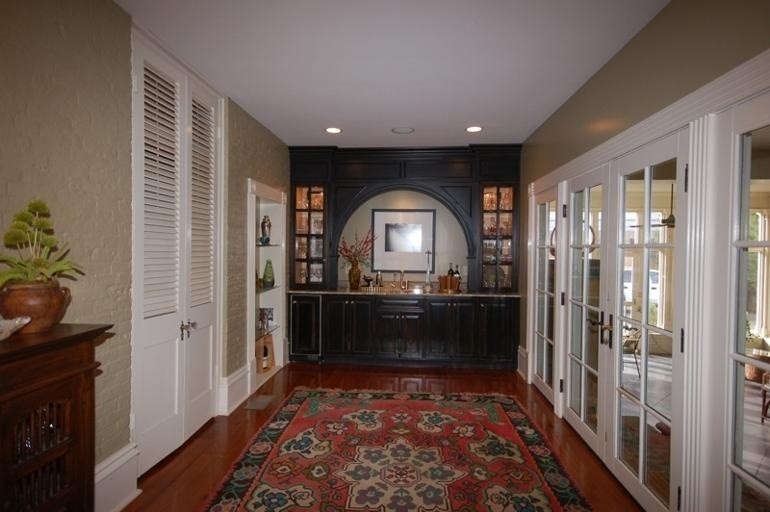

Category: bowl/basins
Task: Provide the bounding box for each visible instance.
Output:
[260,237,270,245]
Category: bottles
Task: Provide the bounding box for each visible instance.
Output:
[261,215,272,238]
[263,345,269,369]
[376,270,382,286]
[447,263,461,278]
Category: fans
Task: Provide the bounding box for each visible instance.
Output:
[628,184,675,228]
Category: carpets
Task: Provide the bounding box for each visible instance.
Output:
[198,385,598,512]
[585,415,770,512]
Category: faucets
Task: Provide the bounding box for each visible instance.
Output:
[391,268,408,289]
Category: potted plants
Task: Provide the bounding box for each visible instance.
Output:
[743,309,770,384]
[0,197,86,335]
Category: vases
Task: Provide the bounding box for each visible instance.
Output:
[348,260,360,289]
[261,216,272,245]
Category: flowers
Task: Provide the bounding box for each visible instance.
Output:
[336,228,380,269]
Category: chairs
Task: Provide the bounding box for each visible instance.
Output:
[621,329,642,380]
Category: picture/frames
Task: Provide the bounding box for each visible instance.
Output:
[370,207,437,274]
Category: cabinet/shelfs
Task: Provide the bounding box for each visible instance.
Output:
[286,292,521,370]
[0,323,116,511]
[469,143,522,293]
[245,177,288,395]
[288,146,336,289]
